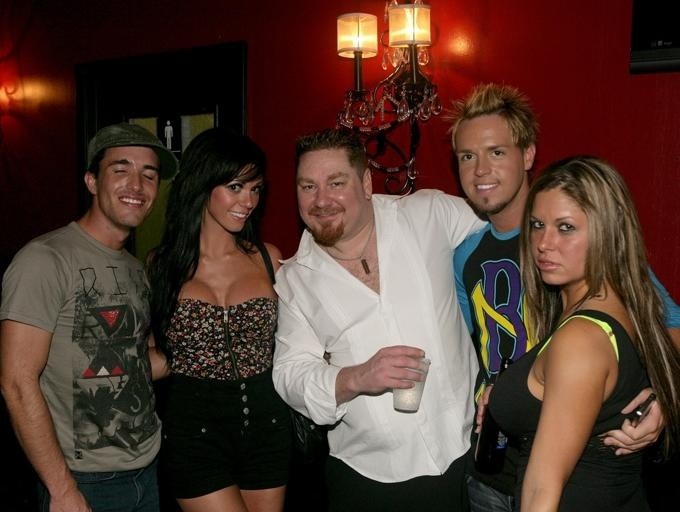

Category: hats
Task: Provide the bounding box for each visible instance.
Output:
[86,123,180,181]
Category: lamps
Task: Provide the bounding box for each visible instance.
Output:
[338,2,441,197]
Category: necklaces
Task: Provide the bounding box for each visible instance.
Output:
[324,213,375,276]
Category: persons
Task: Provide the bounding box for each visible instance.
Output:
[439,79,679,511]
[0,122,172,512]
[144,127,296,511]
[489,154,680,512]
[270,127,493,512]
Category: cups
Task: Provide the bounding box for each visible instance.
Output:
[391,357,431,413]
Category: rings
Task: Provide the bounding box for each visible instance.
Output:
[486,383,494,388]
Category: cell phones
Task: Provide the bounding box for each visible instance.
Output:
[634,393,657,423]
